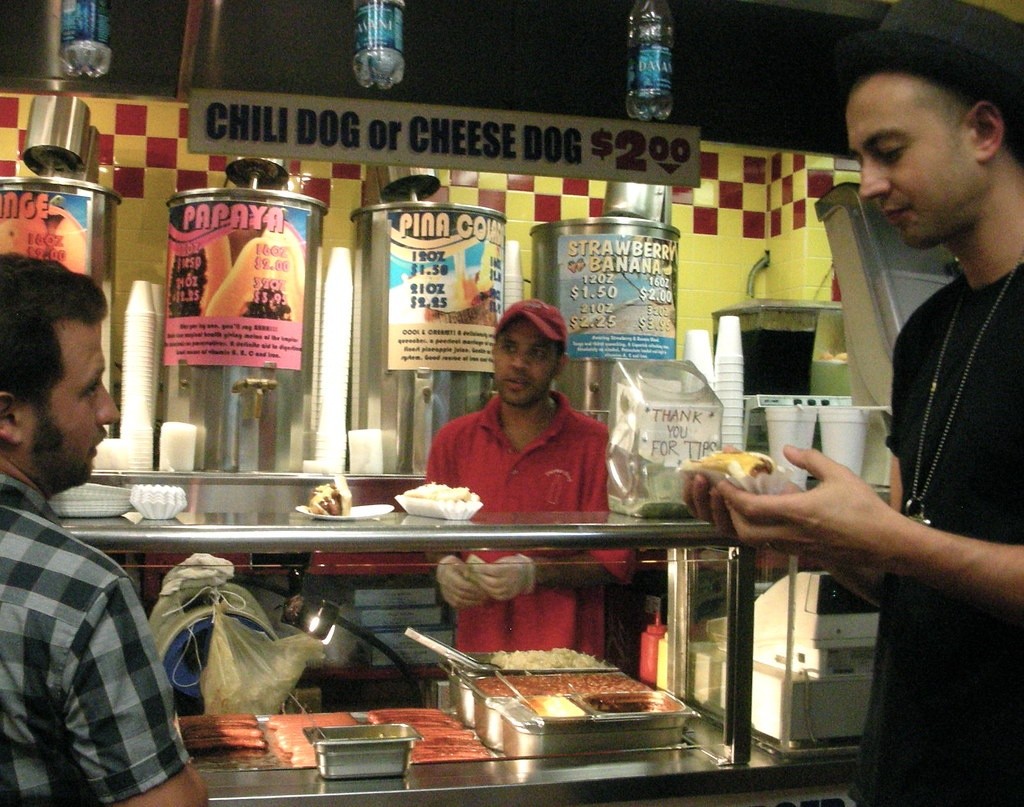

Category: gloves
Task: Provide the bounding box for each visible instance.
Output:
[435,553,536,609]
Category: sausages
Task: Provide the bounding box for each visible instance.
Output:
[178,707,492,767]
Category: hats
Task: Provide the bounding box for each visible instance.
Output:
[840,0,1024,121]
[497,299,568,347]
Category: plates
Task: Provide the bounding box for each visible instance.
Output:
[45,482,132,517]
[291,504,396,520]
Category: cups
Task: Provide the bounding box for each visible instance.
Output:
[686,316,741,457]
[764,404,870,492]
[316,246,387,475]
[90,280,197,473]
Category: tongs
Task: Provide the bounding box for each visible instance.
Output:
[404,627,501,670]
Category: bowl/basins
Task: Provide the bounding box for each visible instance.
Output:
[395,496,484,520]
[130,485,185,518]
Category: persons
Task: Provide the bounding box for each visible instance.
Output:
[427,298,634,680]
[0,254,209,807]
[681,0,1024,807]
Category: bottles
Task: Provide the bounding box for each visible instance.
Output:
[625,0,673,120]
[639,612,669,692]
[351,0,405,89]
[60,0,111,78]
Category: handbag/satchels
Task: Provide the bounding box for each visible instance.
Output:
[199,603,324,715]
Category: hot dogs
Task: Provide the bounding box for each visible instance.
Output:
[310,474,352,518]
[680,451,775,483]
[402,481,482,504]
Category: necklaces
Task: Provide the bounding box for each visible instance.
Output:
[904,243,1024,528]
